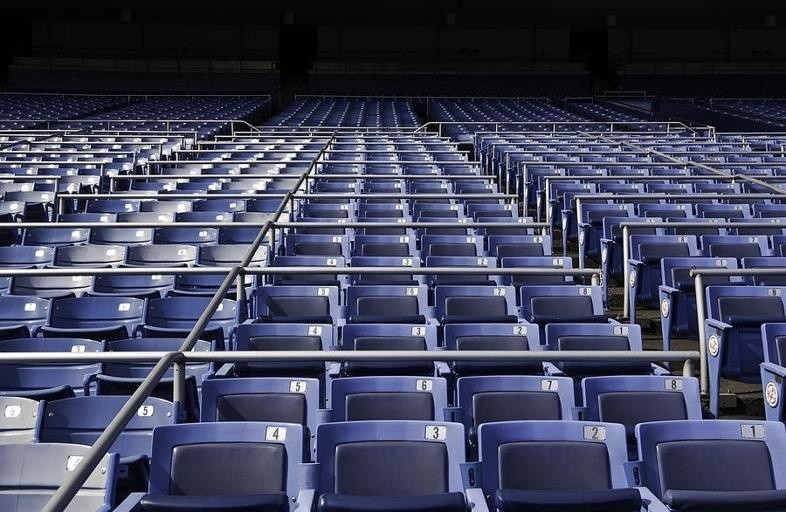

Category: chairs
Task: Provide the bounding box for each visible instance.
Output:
[0,95,786,512]
